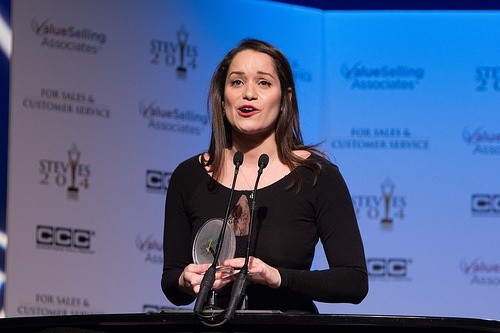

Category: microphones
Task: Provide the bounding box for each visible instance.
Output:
[223,152,270,320]
[195,151,245,313]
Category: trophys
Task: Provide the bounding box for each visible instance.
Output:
[191,215,247,283]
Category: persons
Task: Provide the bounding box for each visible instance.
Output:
[160,34,369,315]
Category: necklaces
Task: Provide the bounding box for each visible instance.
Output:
[231,147,280,202]
